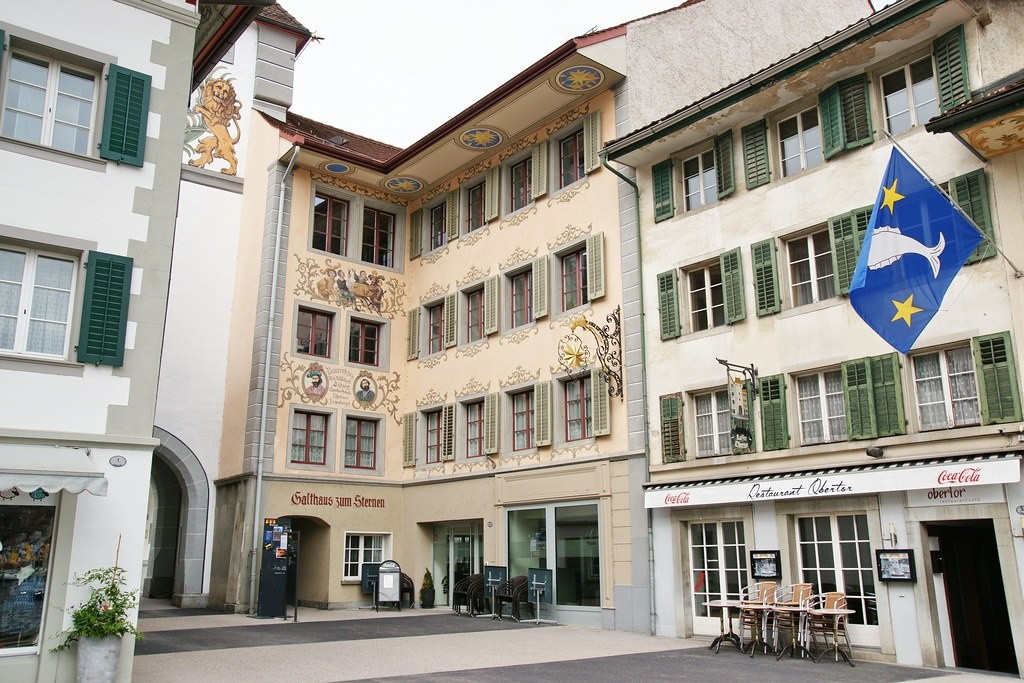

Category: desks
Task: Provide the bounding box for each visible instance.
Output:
[735,604,779,659]
[702,599,743,654]
[711,599,750,654]
[808,609,857,667]
[771,607,817,661]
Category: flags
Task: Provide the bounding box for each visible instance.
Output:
[850,147,984,354]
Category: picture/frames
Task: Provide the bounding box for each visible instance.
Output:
[875,549,918,584]
[749,550,783,581]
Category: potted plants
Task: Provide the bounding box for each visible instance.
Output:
[420,568,435,608]
[40,564,146,683]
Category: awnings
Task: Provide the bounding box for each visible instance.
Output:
[644,453,1022,507]
[0,443,109,496]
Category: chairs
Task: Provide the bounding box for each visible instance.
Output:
[451,573,491,617]
[739,581,855,659]
[393,572,415,609]
[495,575,536,623]
